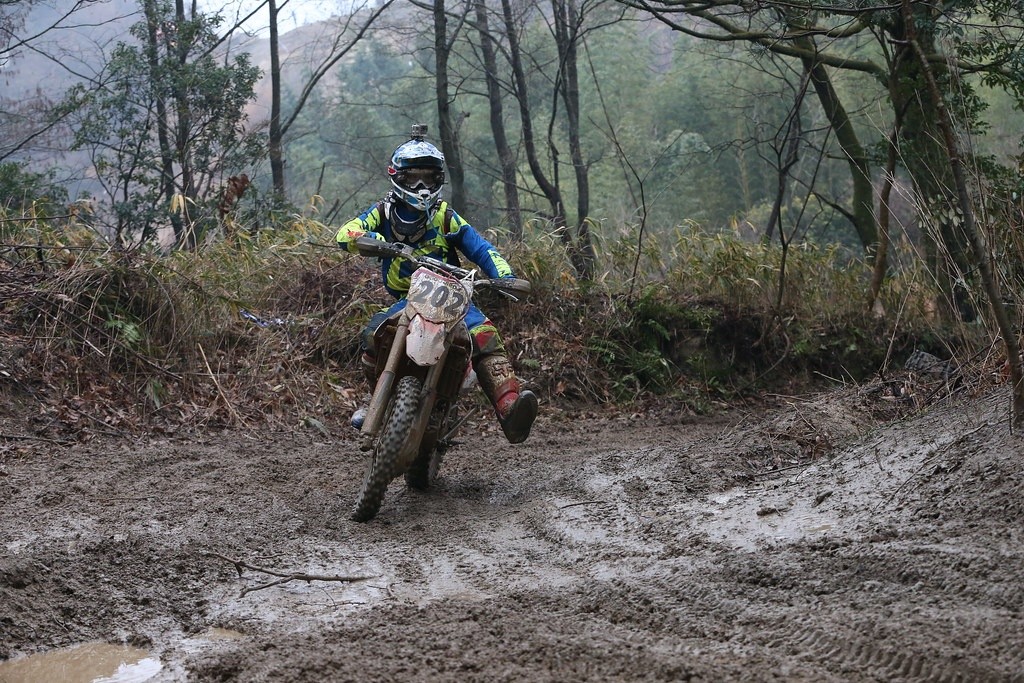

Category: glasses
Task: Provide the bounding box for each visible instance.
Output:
[388,167,442,189]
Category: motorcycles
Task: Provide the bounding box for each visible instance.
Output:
[352,238,531,520]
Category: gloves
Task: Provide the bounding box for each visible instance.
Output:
[363,232,386,242]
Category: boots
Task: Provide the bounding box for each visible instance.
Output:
[475,355,538,444]
[351,352,379,430]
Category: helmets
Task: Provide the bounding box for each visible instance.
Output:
[387,123,446,211]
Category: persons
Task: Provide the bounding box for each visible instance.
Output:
[336,138,538,443]
